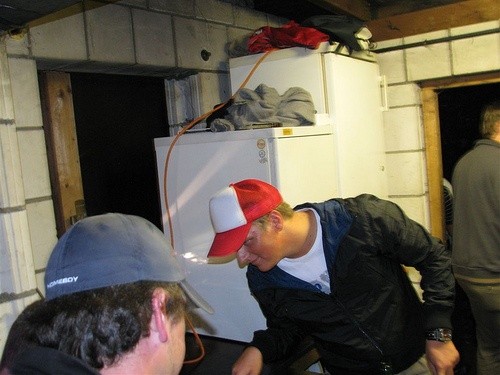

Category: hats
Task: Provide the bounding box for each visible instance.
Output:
[206,178,283,265]
[44,213,216,315]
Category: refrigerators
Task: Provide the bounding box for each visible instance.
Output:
[229,41,388,200]
[154,126,338,373]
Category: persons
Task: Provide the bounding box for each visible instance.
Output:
[0,212,214,375]
[451,107,500,375]
[207,178,460,375]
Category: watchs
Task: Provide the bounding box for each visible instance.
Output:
[427,329,453,343]
